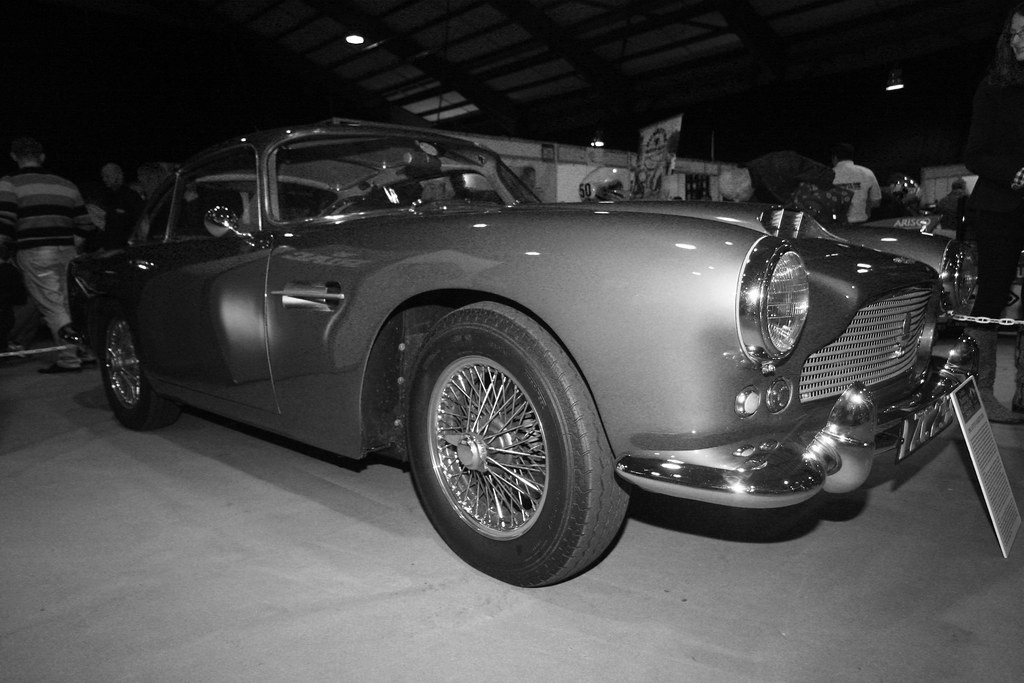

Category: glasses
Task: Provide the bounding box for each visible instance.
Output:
[1008,29,1024,41]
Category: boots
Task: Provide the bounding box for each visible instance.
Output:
[963,328,1024,422]
[1011,326,1024,413]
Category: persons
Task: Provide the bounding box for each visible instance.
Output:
[0,136,143,374]
[832,0,1024,423]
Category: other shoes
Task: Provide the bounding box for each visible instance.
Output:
[36,363,73,373]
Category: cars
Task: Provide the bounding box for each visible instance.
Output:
[65,121,980,588]
